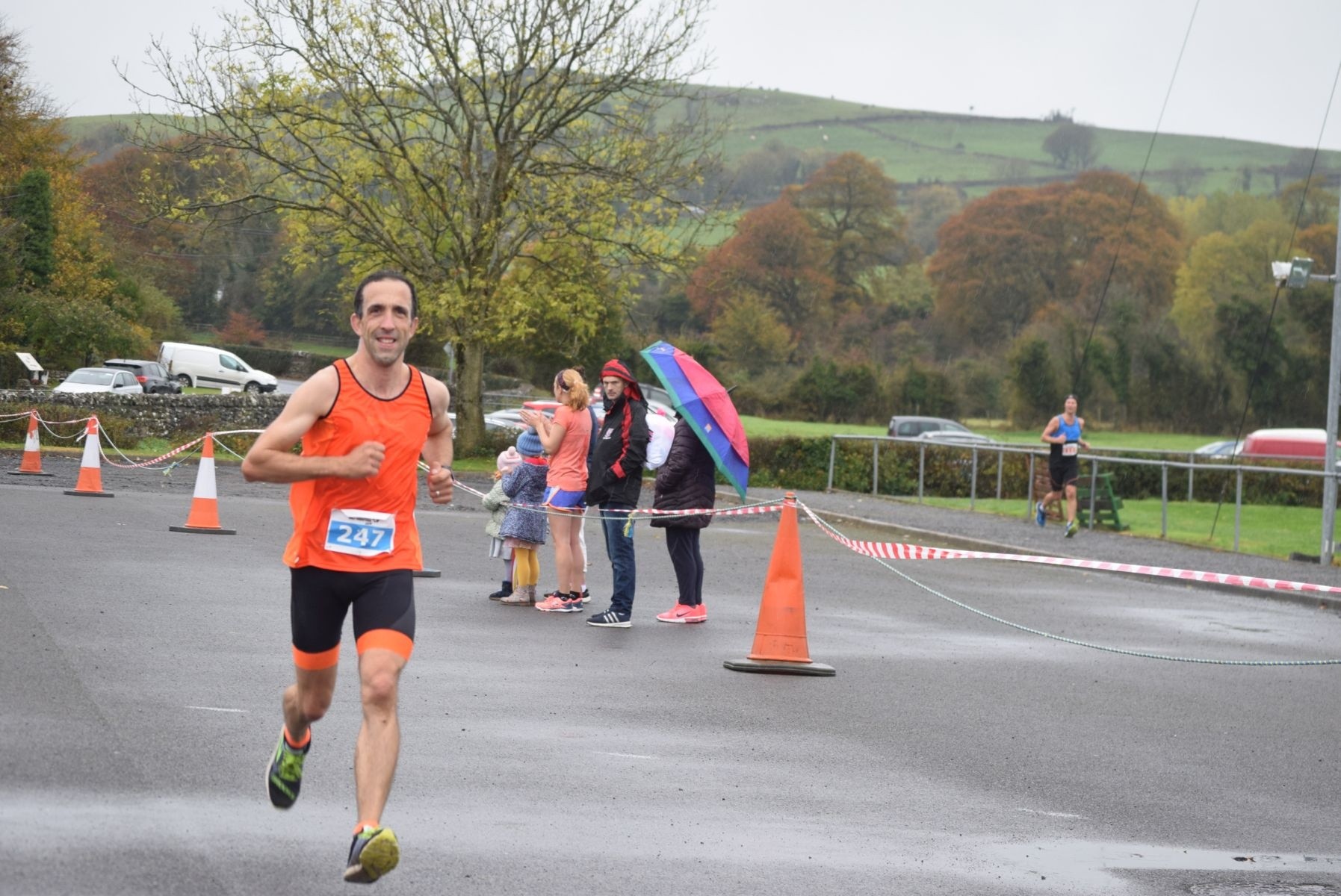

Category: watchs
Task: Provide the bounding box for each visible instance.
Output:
[442,465,455,481]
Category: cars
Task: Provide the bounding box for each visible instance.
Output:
[49,368,143,401]
[916,429,999,487]
[102,359,182,395]
[1194,441,1244,460]
[446,381,676,452]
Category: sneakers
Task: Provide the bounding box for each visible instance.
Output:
[343,821,400,884]
[591,609,609,617]
[266,722,312,810]
[544,594,553,599]
[583,589,592,603]
[700,602,707,621]
[535,592,573,612]
[1064,521,1077,538]
[586,611,632,628]
[572,597,584,612]
[1036,502,1046,525]
[656,599,701,622]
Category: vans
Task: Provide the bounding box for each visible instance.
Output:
[155,341,277,395]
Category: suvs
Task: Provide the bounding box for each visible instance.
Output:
[888,416,971,439]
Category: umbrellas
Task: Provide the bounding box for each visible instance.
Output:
[636,339,751,506]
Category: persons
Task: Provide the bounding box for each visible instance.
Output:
[498,426,547,607]
[481,447,522,600]
[1034,393,1091,537]
[522,368,593,612]
[239,274,452,884]
[543,404,598,603]
[584,357,649,627]
[648,408,714,623]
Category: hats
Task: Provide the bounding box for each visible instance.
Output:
[497,446,523,475]
[1064,393,1080,406]
[516,427,545,457]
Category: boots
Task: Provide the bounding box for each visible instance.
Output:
[529,586,536,606]
[489,581,513,600]
[500,585,530,606]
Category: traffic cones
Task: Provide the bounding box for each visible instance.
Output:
[64,414,115,497]
[7,409,54,476]
[724,490,837,677]
[169,431,237,534]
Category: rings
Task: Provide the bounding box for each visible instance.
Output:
[436,491,441,497]
